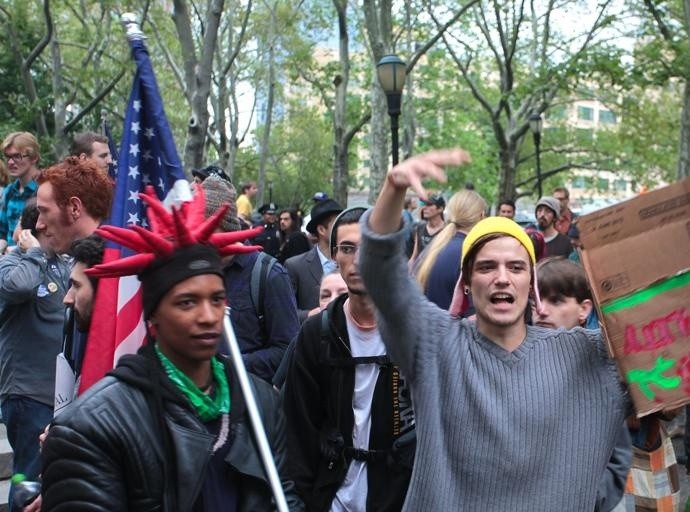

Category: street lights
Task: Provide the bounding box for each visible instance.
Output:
[266,178,274,203]
[528,107,543,199]
[375,53,409,163]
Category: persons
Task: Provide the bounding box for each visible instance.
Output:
[37,234,106,448]
[532,256,661,454]
[0,206,77,508]
[0,132,689,330]
[352,148,634,512]
[42,274,307,510]
[271,206,418,511]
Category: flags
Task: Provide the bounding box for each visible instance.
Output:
[74,31,231,397]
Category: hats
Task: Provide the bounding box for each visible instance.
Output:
[307,200,343,233]
[420,192,446,207]
[84,166,263,320]
[314,192,328,201]
[258,202,278,214]
[536,196,560,217]
[461,216,536,270]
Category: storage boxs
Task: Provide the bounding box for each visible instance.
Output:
[571,176,690,417]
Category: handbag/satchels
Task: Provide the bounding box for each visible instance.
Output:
[611,426,680,512]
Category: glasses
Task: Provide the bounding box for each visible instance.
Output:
[1,155,28,161]
